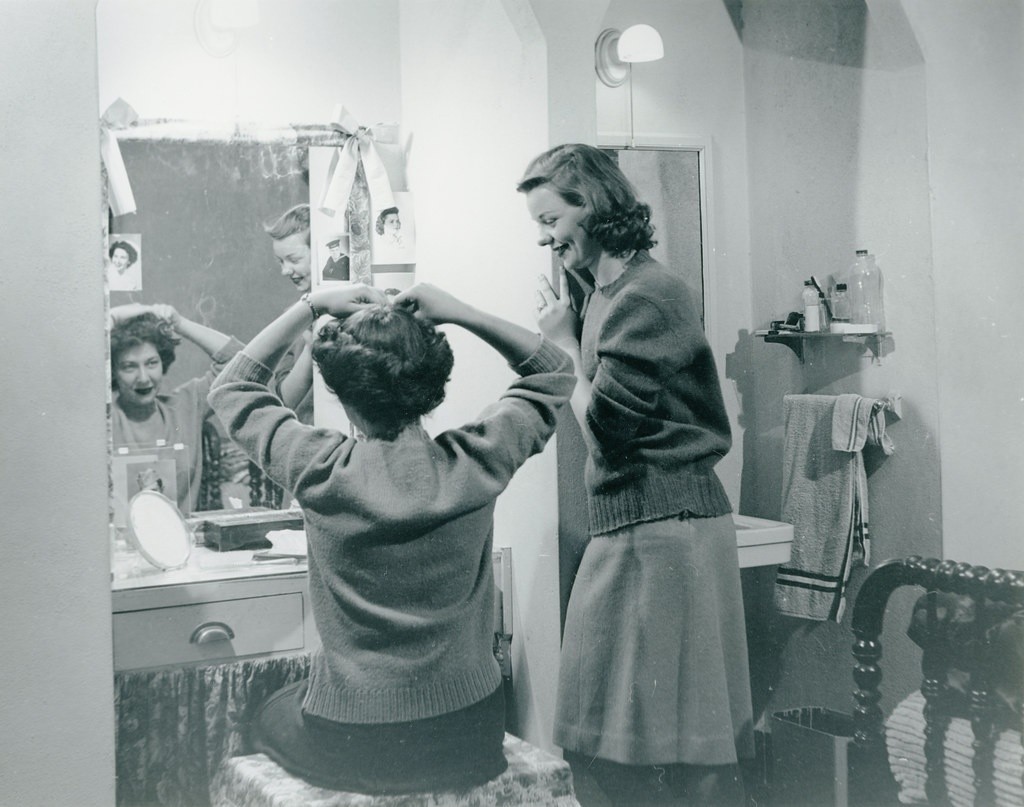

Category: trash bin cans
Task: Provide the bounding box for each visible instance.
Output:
[771,704,855,807]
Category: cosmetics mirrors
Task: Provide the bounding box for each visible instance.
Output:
[126,490,191,568]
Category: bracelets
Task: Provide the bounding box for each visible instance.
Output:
[112,310,119,325]
[300,293,319,320]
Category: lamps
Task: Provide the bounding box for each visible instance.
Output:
[595,22,666,89]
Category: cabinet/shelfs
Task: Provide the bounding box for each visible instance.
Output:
[113,554,514,676]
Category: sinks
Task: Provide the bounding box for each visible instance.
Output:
[731,514,794,568]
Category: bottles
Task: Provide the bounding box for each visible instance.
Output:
[818,291,830,332]
[847,250,879,325]
[801,280,819,334]
[831,283,851,324]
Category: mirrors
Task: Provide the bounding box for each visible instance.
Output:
[594,143,707,336]
[99,132,408,543]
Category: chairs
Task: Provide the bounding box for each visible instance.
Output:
[847,553,1024,807]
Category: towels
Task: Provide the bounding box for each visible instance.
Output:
[771,392,895,625]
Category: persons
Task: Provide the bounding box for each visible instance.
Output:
[375,207,401,264]
[108,303,246,524]
[265,203,314,427]
[517,144,751,807]
[109,241,137,289]
[206,283,577,793]
[322,239,349,282]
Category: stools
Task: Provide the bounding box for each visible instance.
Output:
[208,724,584,807]
